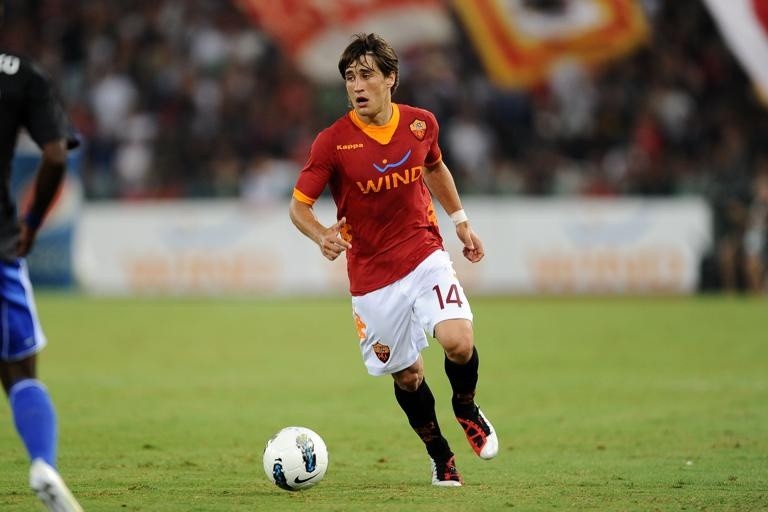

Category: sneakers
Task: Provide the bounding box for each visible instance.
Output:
[453,398,499,460]
[29,458,81,511]
[430,454,464,487]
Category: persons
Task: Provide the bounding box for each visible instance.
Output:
[287,31,501,486]
[0,43,85,512]
[0,0,768,294]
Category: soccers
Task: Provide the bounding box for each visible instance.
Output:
[262,427,328,491]
